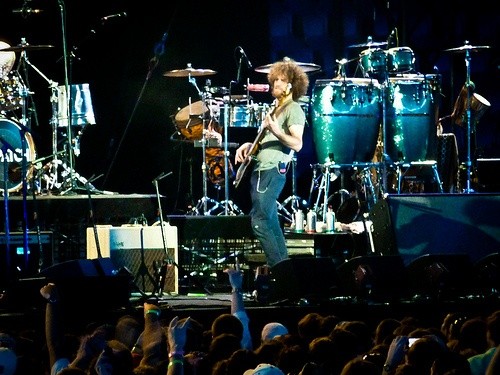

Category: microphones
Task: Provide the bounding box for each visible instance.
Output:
[100,11,127,20]
[238,46,252,68]
[385,28,397,50]
[12,9,40,13]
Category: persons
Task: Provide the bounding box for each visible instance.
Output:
[0,257,500,375]
[251,61,309,268]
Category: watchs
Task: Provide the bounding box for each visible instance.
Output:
[231,288,243,295]
[383,364,396,375]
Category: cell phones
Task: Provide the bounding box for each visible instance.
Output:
[406,337,419,348]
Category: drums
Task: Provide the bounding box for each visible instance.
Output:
[381,70,443,183]
[359,47,389,76]
[386,44,418,73]
[0,117,36,193]
[49,83,98,127]
[175,94,220,142]
[309,75,384,177]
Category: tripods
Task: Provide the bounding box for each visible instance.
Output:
[274,153,316,229]
[35,0,120,196]
[185,73,244,216]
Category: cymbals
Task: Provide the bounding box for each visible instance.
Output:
[252,62,322,75]
[162,67,218,77]
[349,38,390,50]
[444,45,492,52]
[0,42,56,53]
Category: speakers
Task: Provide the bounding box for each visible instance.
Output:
[32,256,133,316]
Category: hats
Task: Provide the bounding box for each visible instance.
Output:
[261,323,289,341]
[243,363,284,375]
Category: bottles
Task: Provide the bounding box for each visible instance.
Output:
[307,209,317,233]
[295,209,304,233]
[326,205,335,234]
